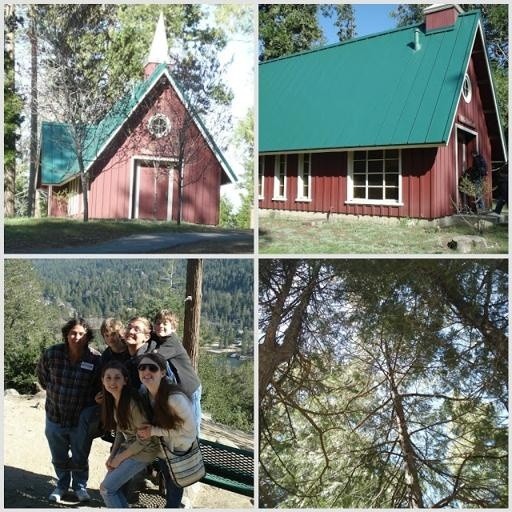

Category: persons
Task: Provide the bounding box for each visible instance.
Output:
[35,317,103,503]
[487,170,508,216]
[89,309,203,508]
[471,149,488,211]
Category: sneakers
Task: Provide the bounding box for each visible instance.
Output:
[49,488,65,503]
[74,488,90,502]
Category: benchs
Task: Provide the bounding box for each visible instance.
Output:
[196,437,254,498]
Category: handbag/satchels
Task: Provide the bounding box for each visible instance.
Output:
[158,437,206,489]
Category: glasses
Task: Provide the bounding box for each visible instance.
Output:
[137,363,160,373]
